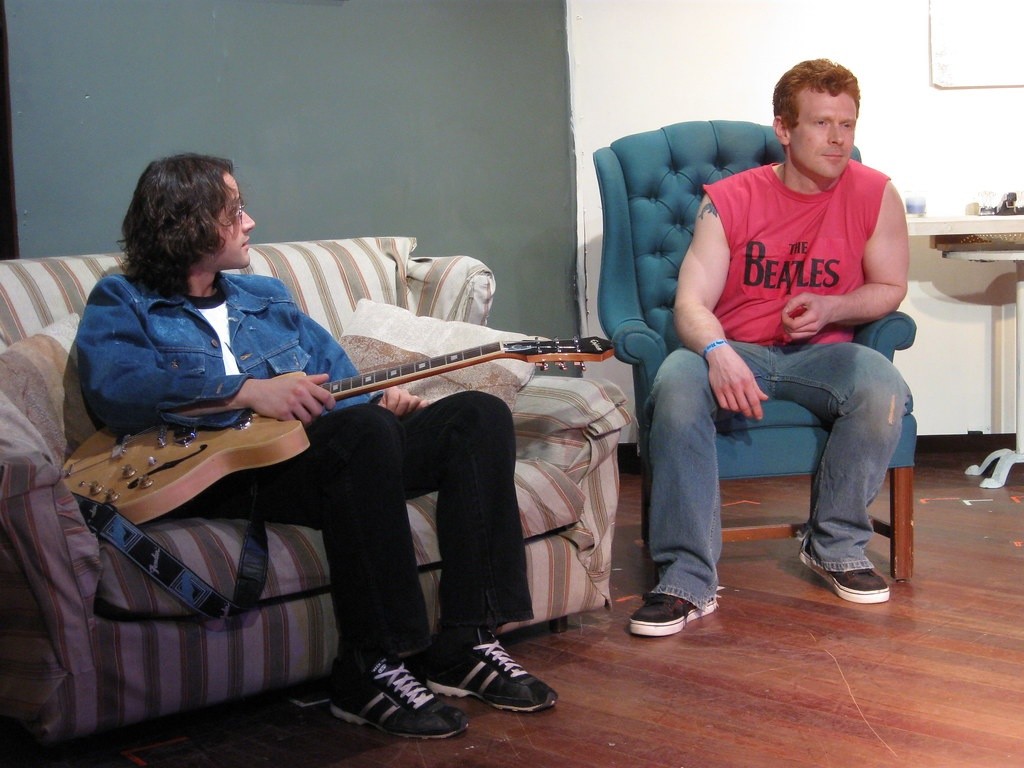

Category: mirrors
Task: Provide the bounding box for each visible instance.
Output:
[929,0,1024,89]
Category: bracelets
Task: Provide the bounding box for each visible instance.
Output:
[703,339,730,358]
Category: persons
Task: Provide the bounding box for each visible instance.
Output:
[80,150,560,740]
[626,57,913,639]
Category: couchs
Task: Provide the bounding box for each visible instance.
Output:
[0,234,633,747]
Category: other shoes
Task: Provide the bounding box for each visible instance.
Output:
[630,593,716,636]
[799,535,890,604]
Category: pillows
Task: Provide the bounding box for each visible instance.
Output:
[0,311,80,472]
[336,298,553,413]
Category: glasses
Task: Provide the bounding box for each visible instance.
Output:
[206,204,244,226]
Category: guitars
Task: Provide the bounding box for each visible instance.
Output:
[62,336,615,526]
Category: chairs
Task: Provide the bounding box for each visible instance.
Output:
[591,120,917,582]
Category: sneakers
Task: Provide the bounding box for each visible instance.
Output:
[331,650,469,740]
[426,624,558,713]
